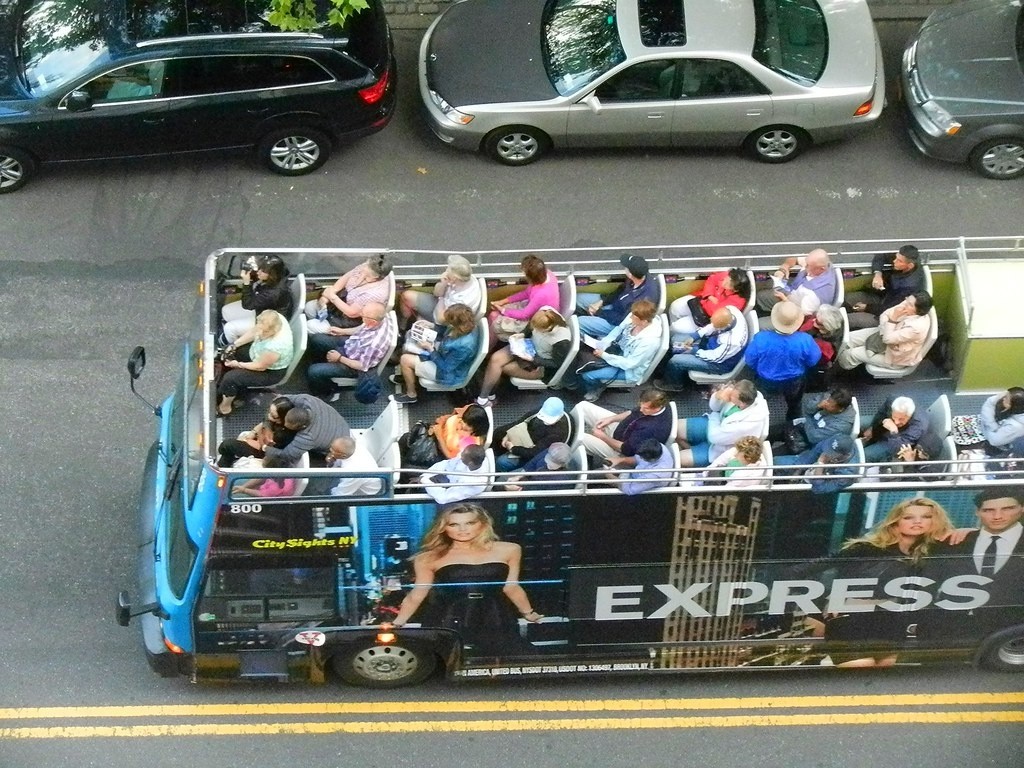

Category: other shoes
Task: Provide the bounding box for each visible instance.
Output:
[482,399,498,407]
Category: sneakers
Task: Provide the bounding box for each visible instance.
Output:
[388,393,417,403]
[653,379,685,392]
[552,382,579,390]
[583,387,606,400]
[390,374,405,385]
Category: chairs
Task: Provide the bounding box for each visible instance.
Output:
[618,62,701,98]
[148,61,165,95]
[236,266,959,496]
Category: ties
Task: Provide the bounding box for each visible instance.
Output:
[980,536,1000,579]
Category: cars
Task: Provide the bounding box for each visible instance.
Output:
[893,0,1024,180]
[416,1,890,165]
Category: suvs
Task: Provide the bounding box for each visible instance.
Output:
[1,2,403,197]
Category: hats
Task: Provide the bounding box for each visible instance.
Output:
[823,434,854,456]
[536,396,565,423]
[770,301,804,334]
[544,441,571,469]
[620,253,647,277]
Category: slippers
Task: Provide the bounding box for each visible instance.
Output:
[215,406,230,416]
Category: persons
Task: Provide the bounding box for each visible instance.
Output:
[382,499,546,666]
[217,394,576,505]
[469,245,933,403]
[676,378,1024,486]
[579,389,674,494]
[215,253,481,419]
[780,497,979,668]
[916,487,1024,650]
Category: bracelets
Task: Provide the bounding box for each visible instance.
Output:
[390,622,402,627]
[520,607,534,616]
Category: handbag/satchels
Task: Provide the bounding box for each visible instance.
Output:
[688,295,711,326]
[785,423,811,454]
[866,333,887,353]
[505,421,535,458]
[574,344,623,374]
[398,421,442,465]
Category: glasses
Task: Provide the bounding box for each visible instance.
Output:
[269,409,281,419]
[378,254,385,268]
[916,445,929,457]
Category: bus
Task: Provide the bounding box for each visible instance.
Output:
[112,243,1024,692]
